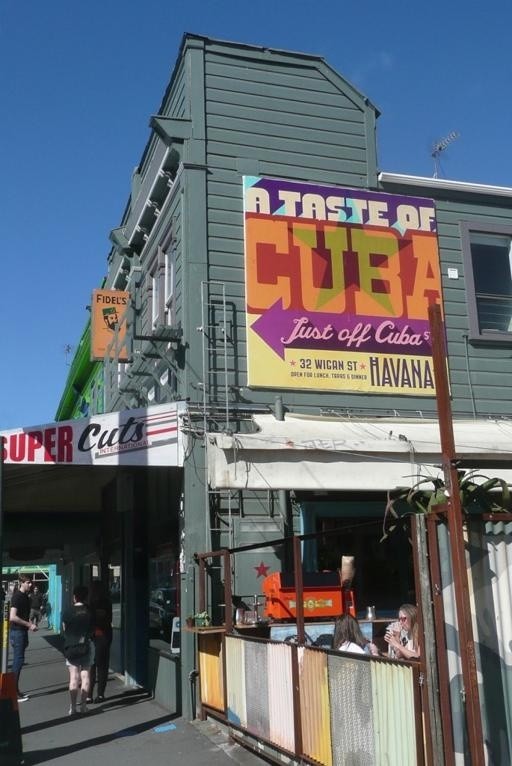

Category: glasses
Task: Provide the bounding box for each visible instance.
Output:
[397,617,408,624]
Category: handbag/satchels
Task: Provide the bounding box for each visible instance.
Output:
[62,636,90,660]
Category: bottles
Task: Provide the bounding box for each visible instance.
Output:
[366,606,377,621]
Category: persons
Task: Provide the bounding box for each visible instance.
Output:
[85,579,113,704]
[27,583,44,631]
[8,572,40,705]
[332,611,380,657]
[59,585,95,717]
[43,588,51,628]
[383,603,422,663]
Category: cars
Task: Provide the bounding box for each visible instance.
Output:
[148,588,177,633]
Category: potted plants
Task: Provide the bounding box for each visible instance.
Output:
[382,464,508,543]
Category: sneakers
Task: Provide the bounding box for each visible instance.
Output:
[68,707,89,716]
[86,696,105,703]
[17,694,30,702]
